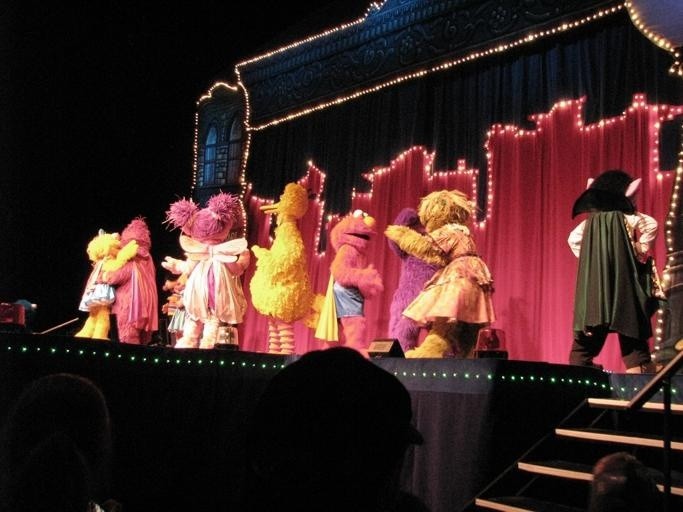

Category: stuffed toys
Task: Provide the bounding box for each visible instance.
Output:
[74,182,496,360]
[563,167,660,374]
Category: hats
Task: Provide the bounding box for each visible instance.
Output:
[256,350,422,446]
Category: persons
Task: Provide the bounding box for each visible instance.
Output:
[189,344,434,512]
[592,450,666,512]
[0,371,122,511]
